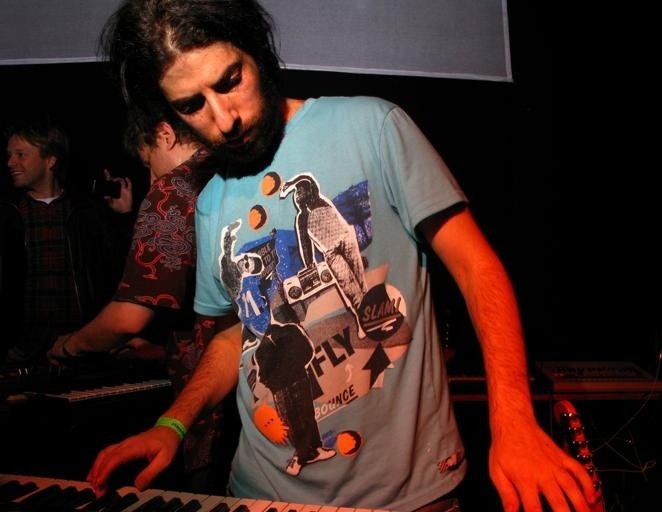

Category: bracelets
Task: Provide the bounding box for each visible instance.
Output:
[152,416,187,441]
[60,333,75,357]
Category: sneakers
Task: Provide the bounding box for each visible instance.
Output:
[356,321,368,340]
[283,453,304,478]
[304,447,337,464]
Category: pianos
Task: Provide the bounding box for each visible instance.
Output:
[0,473,392,512]
[12,358,172,415]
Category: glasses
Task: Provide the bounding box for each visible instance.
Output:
[244,255,250,274]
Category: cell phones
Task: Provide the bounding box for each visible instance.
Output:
[91,178,121,199]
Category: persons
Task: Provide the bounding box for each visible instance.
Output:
[0,114,141,357]
[280,173,369,315]
[41,86,241,497]
[219,219,265,306]
[81,0,604,512]
